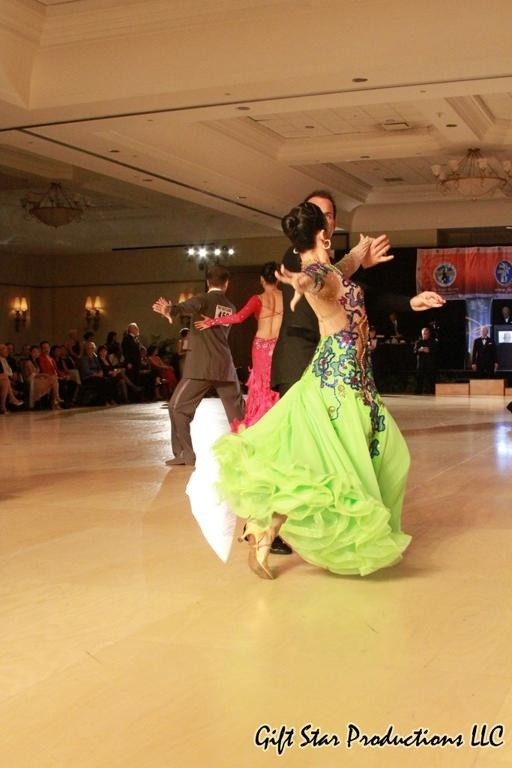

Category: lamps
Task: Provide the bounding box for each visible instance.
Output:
[430,147,511,201]
[14,296,29,332]
[85,295,102,331]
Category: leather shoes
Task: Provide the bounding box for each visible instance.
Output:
[10,399,23,406]
[242,524,292,554]
[52,399,63,409]
[166,459,194,464]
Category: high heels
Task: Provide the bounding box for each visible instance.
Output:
[239,521,274,580]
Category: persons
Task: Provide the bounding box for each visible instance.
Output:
[410,326,438,395]
[469,323,499,379]
[499,306,511,325]
[193,256,283,432]
[150,265,247,466]
[383,310,411,343]
[202,196,415,580]
[0,322,196,416]
[366,325,377,351]
[242,188,446,555]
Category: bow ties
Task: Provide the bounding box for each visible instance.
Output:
[482,337,487,340]
